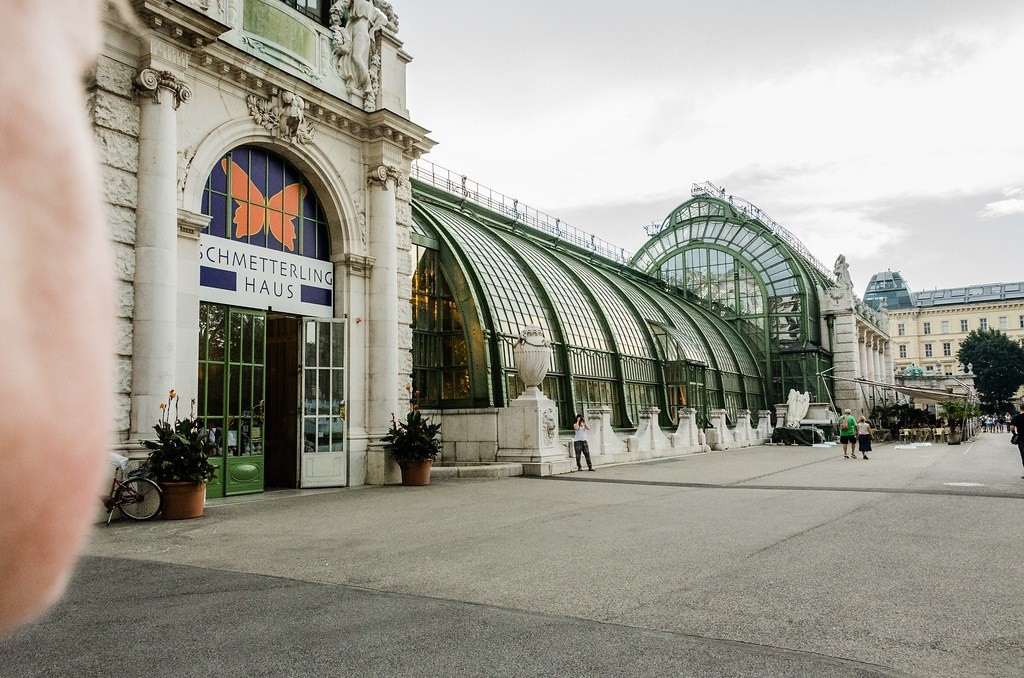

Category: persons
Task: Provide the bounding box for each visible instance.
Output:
[989,415,995,433]
[836,408,858,459]
[993,413,999,433]
[984,416,990,432]
[857,415,875,460]
[1011,401,1024,479]
[1004,412,1012,433]
[998,414,1004,433]
[573,413,595,471]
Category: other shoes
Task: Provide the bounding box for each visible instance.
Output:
[844,455,849,459]
[589,467,595,471]
[983,430,1010,433]
[578,468,582,471]
[851,454,857,459]
[862,455,868,459]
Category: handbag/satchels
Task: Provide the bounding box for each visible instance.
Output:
[1010,434,1020,444]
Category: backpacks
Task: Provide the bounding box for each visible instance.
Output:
[840,415,849,430]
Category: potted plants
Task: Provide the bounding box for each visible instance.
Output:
[936,399,975,444]
[379,386,443,485]
[128,390,220,519]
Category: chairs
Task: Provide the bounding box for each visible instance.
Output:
[899,426,960,443]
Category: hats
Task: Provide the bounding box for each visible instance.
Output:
[844,408,851,413]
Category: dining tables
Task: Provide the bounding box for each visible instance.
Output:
[870,429,891,442]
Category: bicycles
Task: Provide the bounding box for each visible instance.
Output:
[100,453,166,527]
